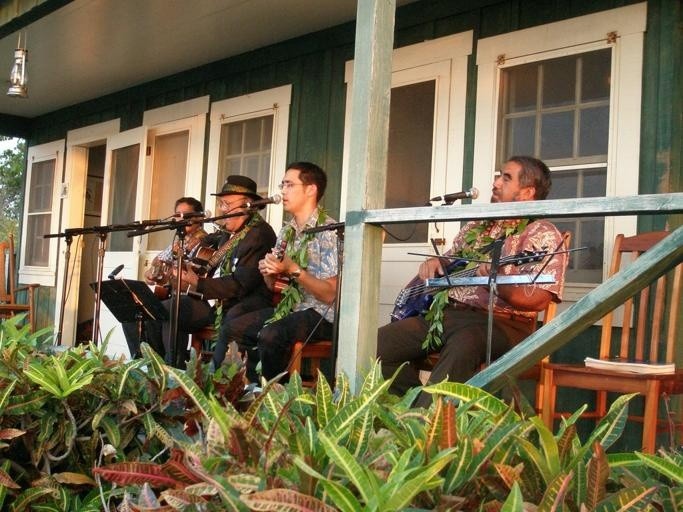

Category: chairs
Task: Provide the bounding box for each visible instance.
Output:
[286,329,336,388]
[541,228,683,454]
[193,326,216,364]
[423,231,576,373]
[1,228,37,335]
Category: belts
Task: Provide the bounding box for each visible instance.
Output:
[447,300,534,329]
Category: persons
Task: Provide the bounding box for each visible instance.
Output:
[121,196,215,370]
[163,176,276,372]
[374,155,569,414]
[215,162,339,387]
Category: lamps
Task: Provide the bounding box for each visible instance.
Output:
[5,21,32,100]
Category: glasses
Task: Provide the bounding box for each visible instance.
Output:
[216,196,248,210]
[278,181,315,191]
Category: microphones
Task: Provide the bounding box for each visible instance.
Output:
[426,188,481,202]
[171,209,213,218]
[240,195,282,208]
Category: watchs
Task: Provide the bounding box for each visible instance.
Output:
[290,267,301,279]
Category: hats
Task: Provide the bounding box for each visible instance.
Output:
[210,174,266,212]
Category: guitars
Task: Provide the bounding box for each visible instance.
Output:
[388,244,549,321]
[270,230,291,321]
[153,243,174,300]
[171,245,217,304]
